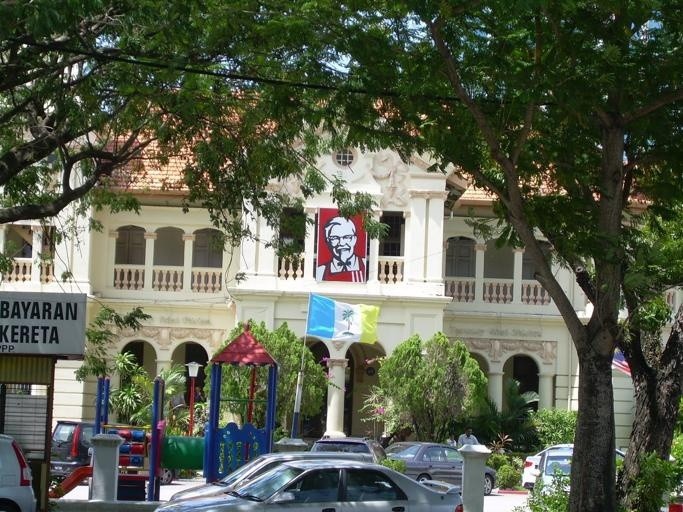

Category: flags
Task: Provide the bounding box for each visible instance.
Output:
[612,351,631,377]
[306,292,380,344]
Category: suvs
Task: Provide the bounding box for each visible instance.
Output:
[165,452,376,500]
[49,421,173,487]
[0,432,35,512]
[310,438,386,467]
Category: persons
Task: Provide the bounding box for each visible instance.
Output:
[456,427,479,448]
[443,431,457,446]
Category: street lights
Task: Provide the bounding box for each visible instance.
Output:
[180,361,202,437]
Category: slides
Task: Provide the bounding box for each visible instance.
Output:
[48,466,93,498]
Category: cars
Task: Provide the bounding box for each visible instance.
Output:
[154,459,465,512]
[386,440,496,498]
[538,449,571,500]
[521,444,627,492]
